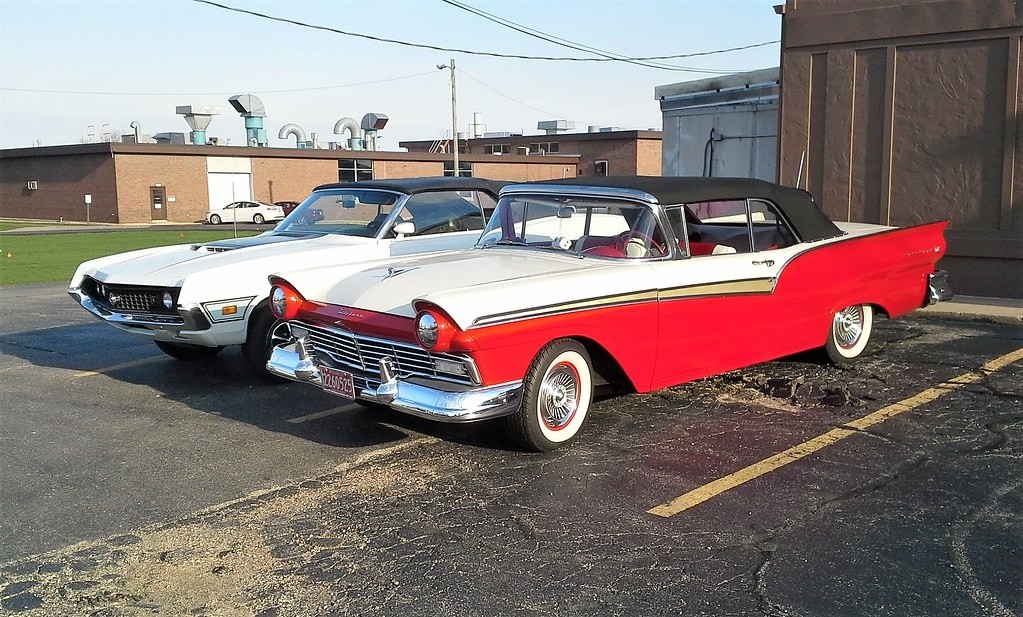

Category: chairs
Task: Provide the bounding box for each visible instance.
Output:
[373,212,463,233]
[575,235,737,258]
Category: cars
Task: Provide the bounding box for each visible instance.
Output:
[67,178,630,363]
[272,202,324,225]
[264,178,956,451]
[206,200,284,225]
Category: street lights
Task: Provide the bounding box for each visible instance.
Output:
[437,58,459,178]
